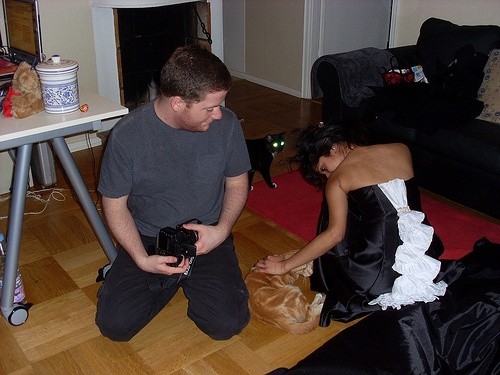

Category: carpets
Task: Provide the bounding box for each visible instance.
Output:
[245,166,500,257]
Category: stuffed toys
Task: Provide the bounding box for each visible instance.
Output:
[1,60,45,119]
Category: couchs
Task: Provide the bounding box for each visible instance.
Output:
[310,16,500,224]
[263,235,500,375]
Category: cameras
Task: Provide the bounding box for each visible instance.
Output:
[156,219,202,268]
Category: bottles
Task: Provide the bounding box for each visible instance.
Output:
[0,233,26,305]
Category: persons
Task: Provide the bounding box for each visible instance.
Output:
[95,45,252,343]
[251,121,449,327]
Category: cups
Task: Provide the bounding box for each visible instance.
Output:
[36,55,79,113]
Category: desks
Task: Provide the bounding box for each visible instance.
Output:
[0,86,130,327]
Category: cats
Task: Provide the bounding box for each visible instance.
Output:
[245,249,326,334]
[244,130,287,192]
